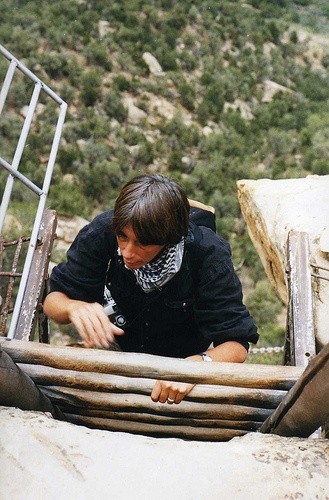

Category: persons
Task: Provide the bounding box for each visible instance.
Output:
[41,170,258,406]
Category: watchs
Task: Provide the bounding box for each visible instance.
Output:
[196,352,212,362]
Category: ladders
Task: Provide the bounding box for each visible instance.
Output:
[0,335,329,443]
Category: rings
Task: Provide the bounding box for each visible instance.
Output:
[167,399,174,404]
